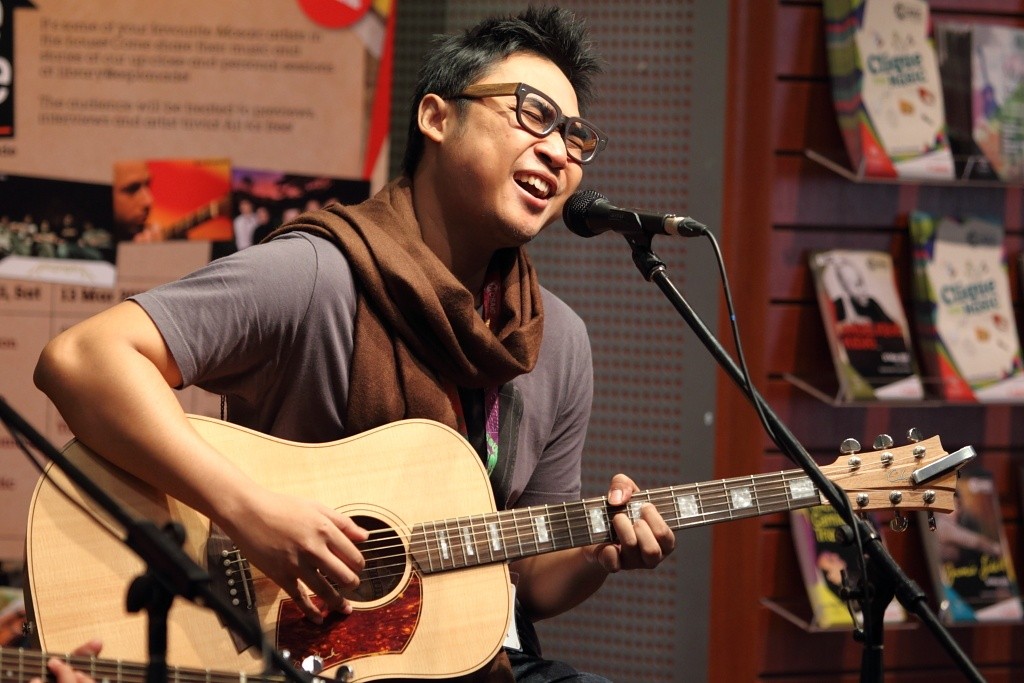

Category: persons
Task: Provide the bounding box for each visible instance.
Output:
[937,491,1001,607]
[0,175,339,270]
[32,0,677,683]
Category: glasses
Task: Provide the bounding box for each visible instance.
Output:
[441,82,609,165]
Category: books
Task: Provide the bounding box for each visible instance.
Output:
[786,0,1024,630]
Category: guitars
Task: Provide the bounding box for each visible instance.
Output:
[22,412,976,683]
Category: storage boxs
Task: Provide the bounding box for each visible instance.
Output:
[0,242,214,574]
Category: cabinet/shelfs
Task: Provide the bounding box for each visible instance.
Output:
[761,146,1024,632]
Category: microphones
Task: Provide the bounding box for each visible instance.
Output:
[563,189,710,239]
